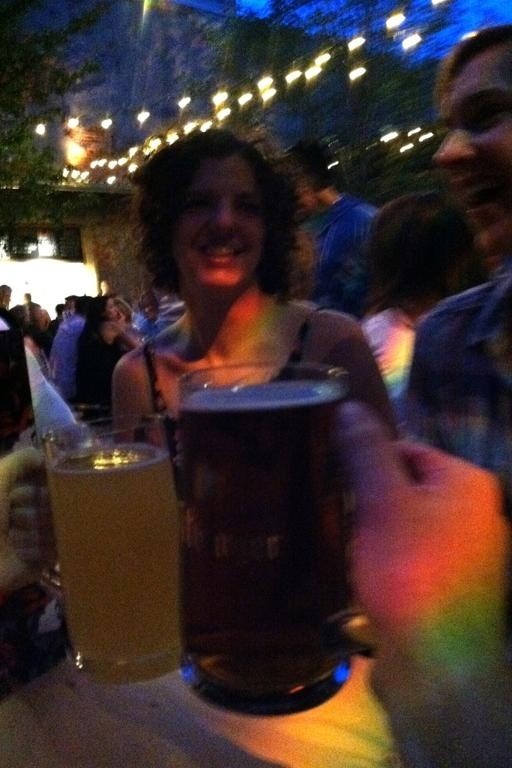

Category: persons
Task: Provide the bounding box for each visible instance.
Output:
[1,21,512,768]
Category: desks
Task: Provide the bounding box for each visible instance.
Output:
[1,653,398,768]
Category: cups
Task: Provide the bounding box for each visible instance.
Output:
[40,410,183,690]
[174,364,421,717]
[27,406,112,594]
